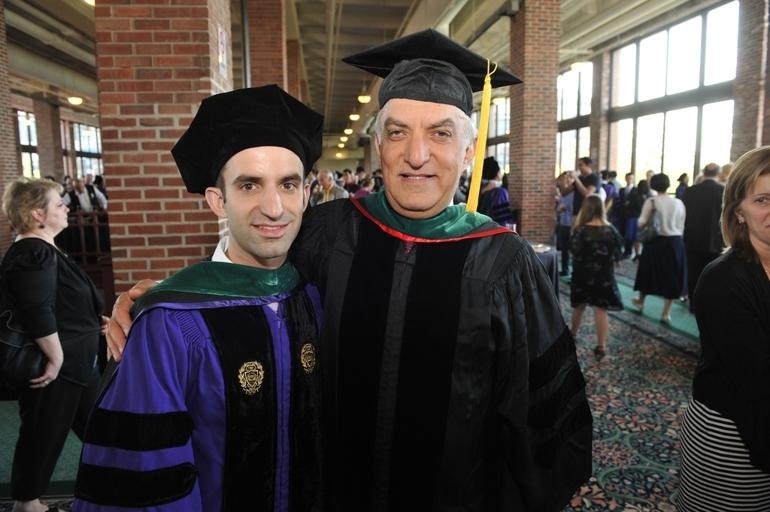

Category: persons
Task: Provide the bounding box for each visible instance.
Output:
[678,162,734,313]
[633,172,686,320]
[69,145,325,512]
[554,157,656,274]
[453,156,510,227]
[0,174,104,512]
[107,99,590,512]
[568,195,625,355]
[675,144,770,512]
[56,176,108,270]
[306,166,383,206]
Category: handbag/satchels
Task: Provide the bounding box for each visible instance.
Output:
[635,222,657,244]
[1,304,48,402]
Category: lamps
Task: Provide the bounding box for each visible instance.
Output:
[333,78,372,162]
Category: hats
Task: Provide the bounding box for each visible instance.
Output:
[340,29,525,216]
[170,84,324,193]
[482,156,499,179]
